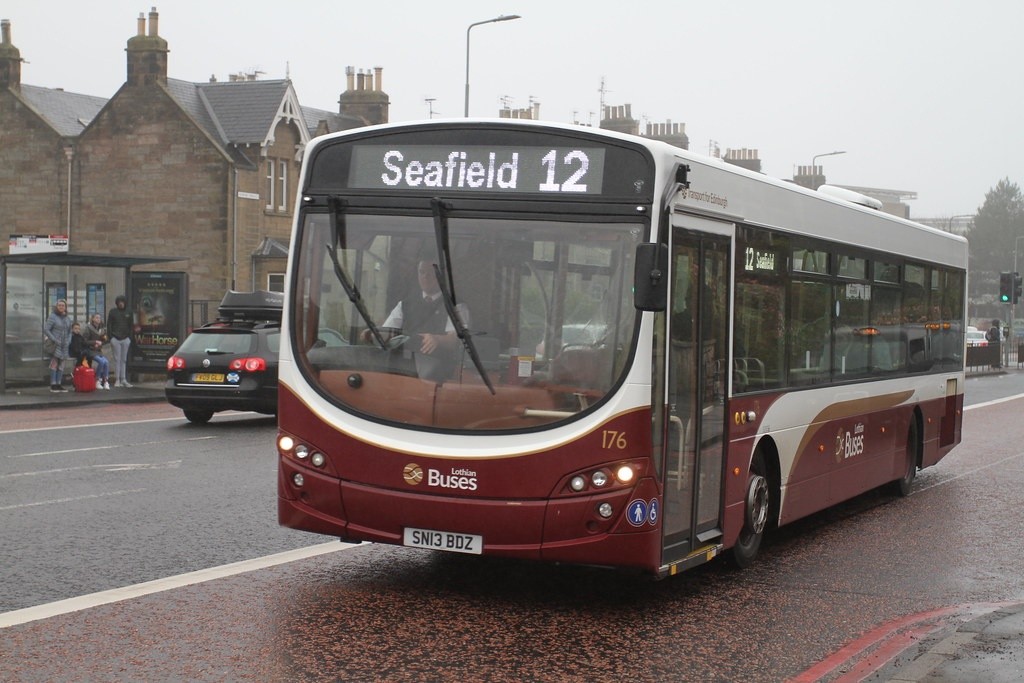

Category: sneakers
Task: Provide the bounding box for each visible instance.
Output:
[121,381,133,388]
[51,385,68,393]
[102,382,110,390]
[96,381,103,389]
[115,380,124,387]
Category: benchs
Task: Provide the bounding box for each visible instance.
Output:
[23,356,77,363]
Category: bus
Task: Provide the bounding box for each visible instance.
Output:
[276,118,970,585]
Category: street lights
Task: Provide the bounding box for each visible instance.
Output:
[465,15,522,119]
[813,152,846,190]
[425,98,437,119]
[949,215,974,234]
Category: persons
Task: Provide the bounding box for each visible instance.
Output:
[43,298,110,393]
[107,295,135,388]
[359,251,470,356]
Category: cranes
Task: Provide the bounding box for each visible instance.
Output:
[597,77,614,119]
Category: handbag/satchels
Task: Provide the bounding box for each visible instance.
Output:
[44,338,57,355]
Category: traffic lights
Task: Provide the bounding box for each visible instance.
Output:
[1013,272,1022,304]
[998,271,1013,304]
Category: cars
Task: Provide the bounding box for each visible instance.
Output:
[967,325,988,348]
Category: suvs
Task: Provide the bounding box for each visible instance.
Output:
[165,290,283,424]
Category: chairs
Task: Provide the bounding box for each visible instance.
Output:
[538,337,606,398]
